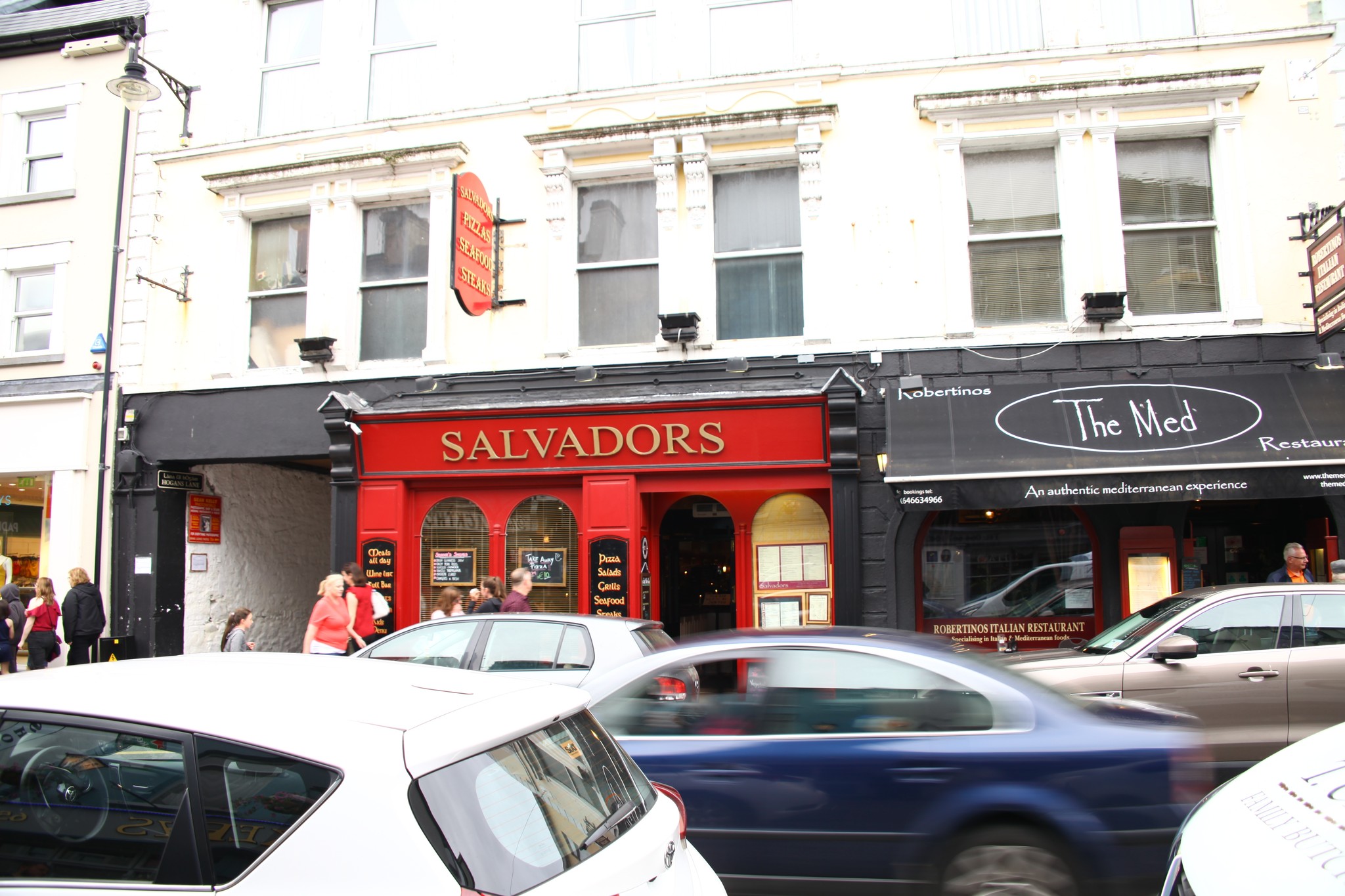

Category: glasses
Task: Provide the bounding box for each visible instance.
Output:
[1289,555,1308,559]
[454,595,464,602]
[67,577,71,580]
[34,584,38,587]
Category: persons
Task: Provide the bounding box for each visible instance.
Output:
[467,576,506,614]
[0,599,14,675]
[341,563,378,652]
[431,586,466,666]
[0,552,12,600]
[1267,542,1320,636]
[499,567,532,613]
[0,582,26,673]
[302,574,350,656]
[17,577,61,671]
[61,568,106,665]
[1330,560,1345,583]
[221,608,255,652]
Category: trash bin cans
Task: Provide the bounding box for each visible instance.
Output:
[98,635,131,661]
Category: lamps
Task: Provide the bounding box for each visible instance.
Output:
[1081,292,1128,332]
[294,337,338,373]
[657,312,701,351]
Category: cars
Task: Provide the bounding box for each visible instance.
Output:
[575,624,1205,896]
[922,546,1095,620]
[1159,718,1345,896]
[914,583,1345,761]
[1,652,728,895]
[349,612,700,736]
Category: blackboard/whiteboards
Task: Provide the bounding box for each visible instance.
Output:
[430,548,478,587]
[518,546,566,587]
[1182,557,1203,591]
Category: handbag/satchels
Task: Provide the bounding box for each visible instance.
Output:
[56,634,62,644]
[371,589,390,619]
[47,641,61,662]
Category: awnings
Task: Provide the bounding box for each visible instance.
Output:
[881,366,1345,514]
